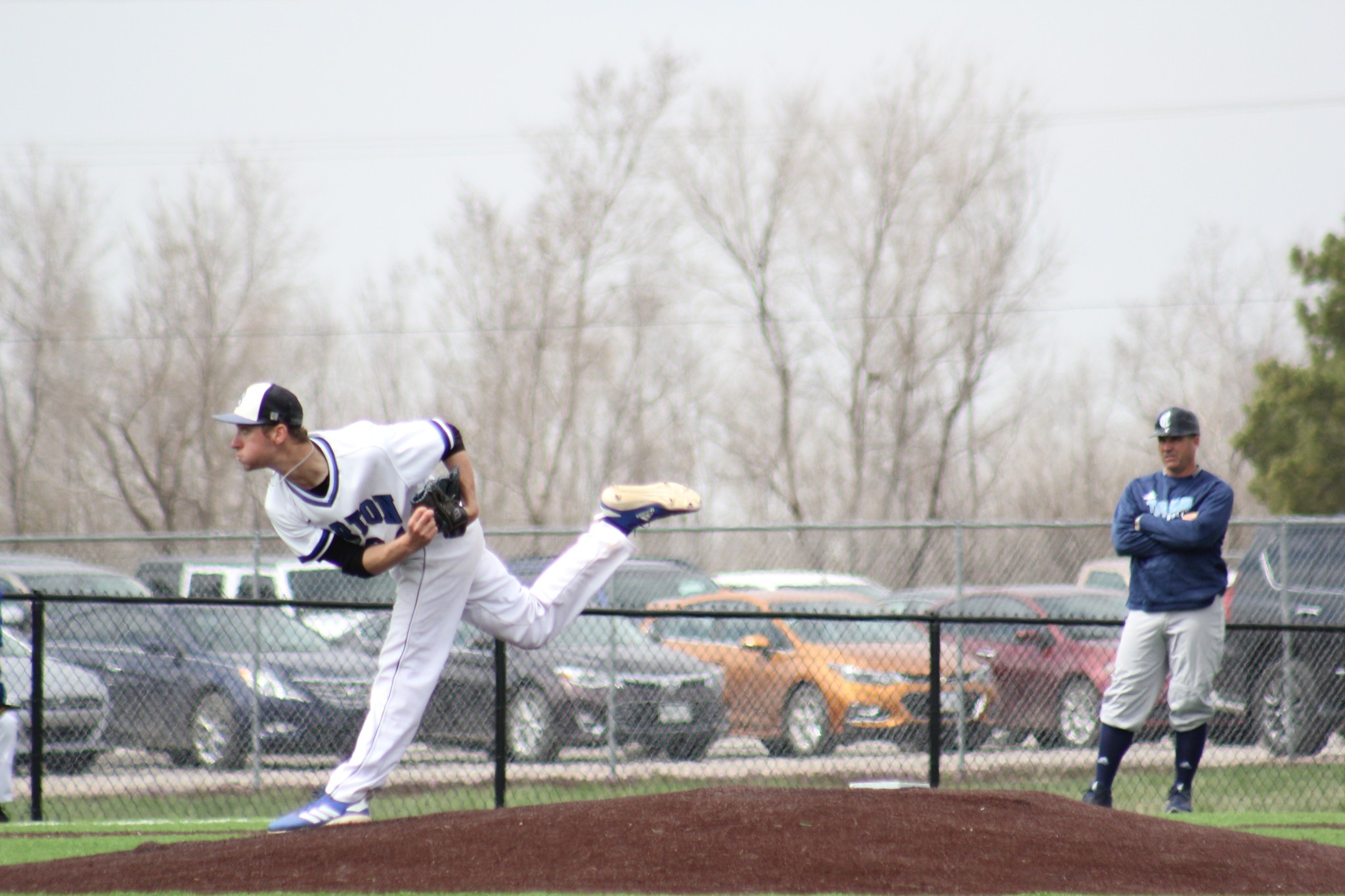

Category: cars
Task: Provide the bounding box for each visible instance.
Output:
[1212,526,1345,758]
[916,585,1174,749]
[0,628,113,775]
[507,554,723,638]
[0,556,152,642]
[333,613,727,762]
[639,589,998,757]
[1078,559,1246,719]
[20,602,377,772]
[677,571,883,596]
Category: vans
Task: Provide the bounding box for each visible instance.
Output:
[131,556,394,640]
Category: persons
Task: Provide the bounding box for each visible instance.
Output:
[212,381,703,835]
[1081,407,1235,812]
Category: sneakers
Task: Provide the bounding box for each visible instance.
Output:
[1080,780,1112,808]
[267,798,371,830]
[601,481,705,525]
[1167,779,1190,814]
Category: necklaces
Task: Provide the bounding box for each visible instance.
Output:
[271,441,314,486]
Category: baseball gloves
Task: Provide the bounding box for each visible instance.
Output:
[409,466,468,539]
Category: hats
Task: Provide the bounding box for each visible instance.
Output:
[213,378,309,433]
[1155,406,1199,440]
